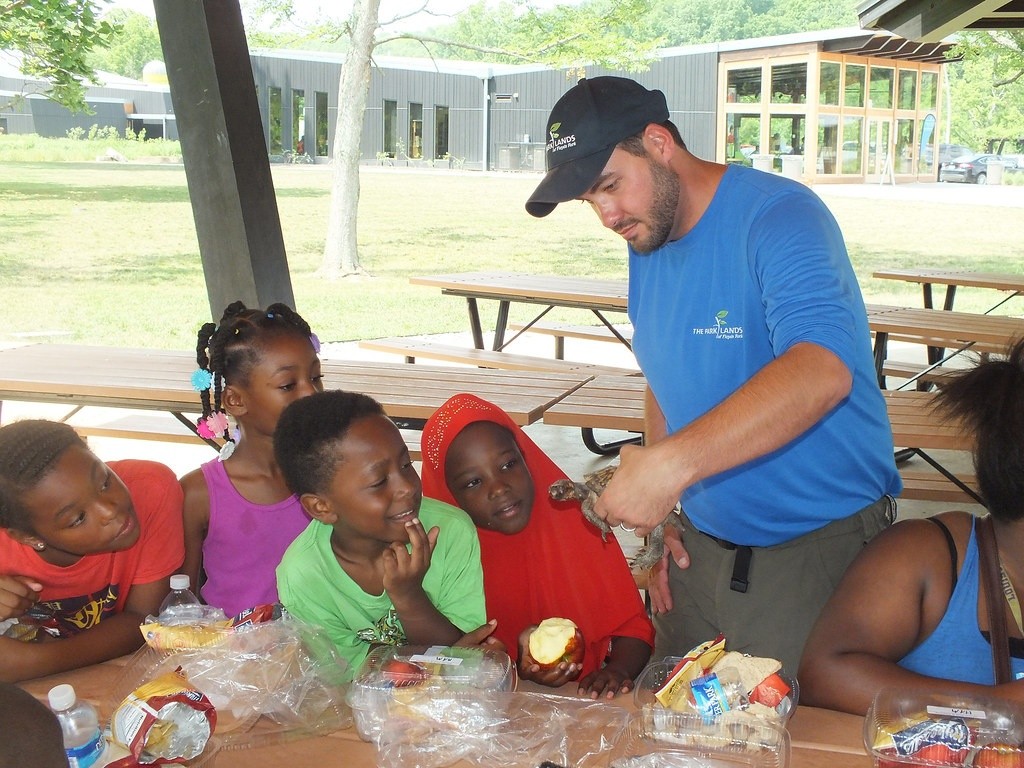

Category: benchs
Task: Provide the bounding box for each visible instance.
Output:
[511,318,636,361]
[61,405,231,449]
[892,470,985,503]
[870,332,1014,366]
[358,337,645,451]
[881,363,970,386]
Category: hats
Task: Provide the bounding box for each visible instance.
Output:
[525,75,670,218]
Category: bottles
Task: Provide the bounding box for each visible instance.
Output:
[160,574,203,622]
[49,683,104,768]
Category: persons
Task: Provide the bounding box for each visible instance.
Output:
[728,125,735,143]
[275,391,507,685]
[0,420,185,684]
[770,133,805,168]
[791,336,1024,717]
[524,75,903,678]
[419,394,657,699]
[177,300,325,619]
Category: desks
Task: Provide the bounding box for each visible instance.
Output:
[1,265,1023,512]
[20,642,1023,767]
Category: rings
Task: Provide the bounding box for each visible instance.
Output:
[620,522,637,532]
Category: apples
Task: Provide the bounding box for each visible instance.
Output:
[387,658,426,687]
[527,616,585,671]
[972,743,1024,768]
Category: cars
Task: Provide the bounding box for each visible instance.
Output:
[940,153,1024,186]
[842,141,876,162]
[926,144,976,165]
